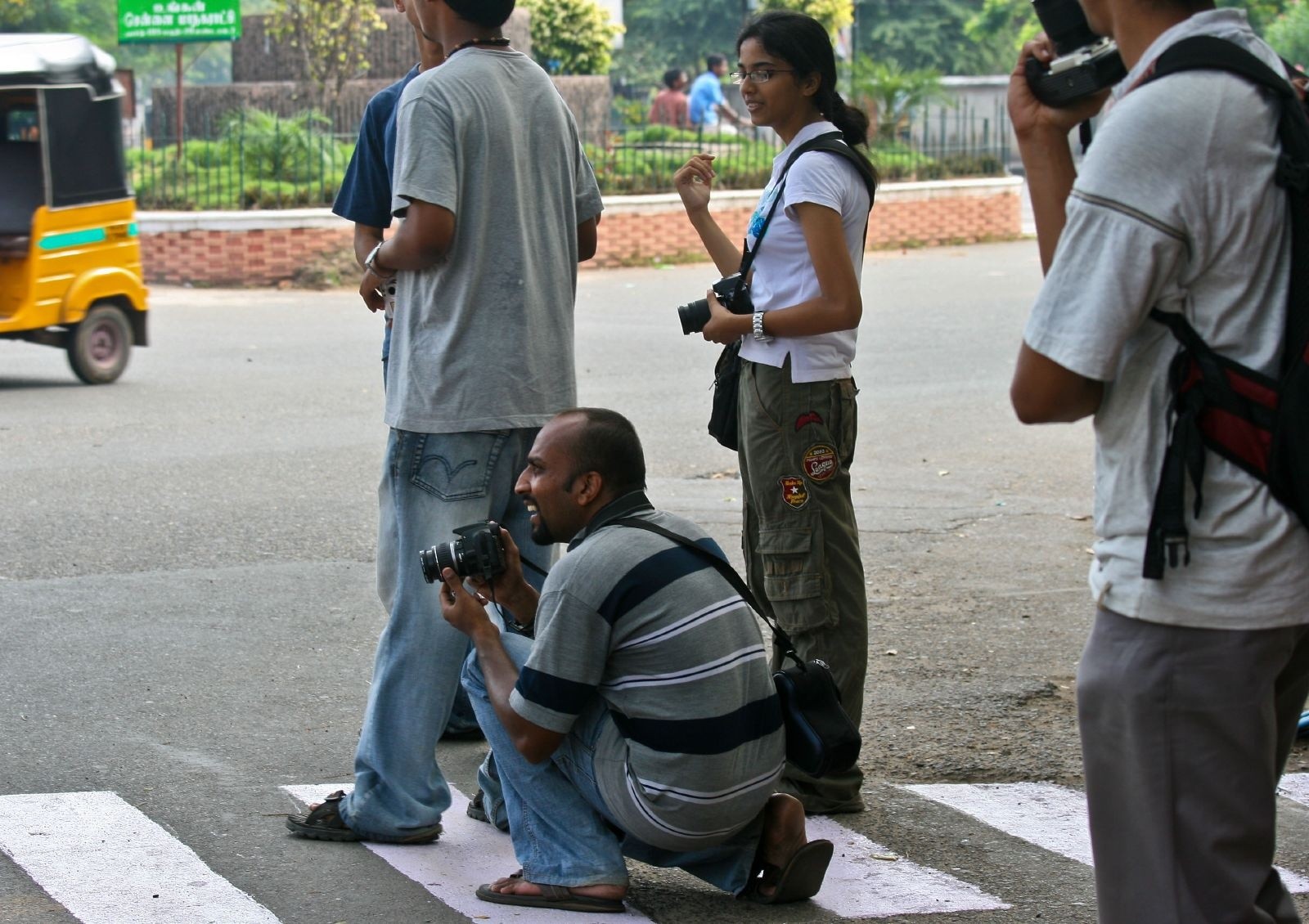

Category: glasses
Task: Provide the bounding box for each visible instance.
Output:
[730,69,793,86]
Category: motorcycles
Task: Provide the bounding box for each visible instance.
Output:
[0,30,151,387]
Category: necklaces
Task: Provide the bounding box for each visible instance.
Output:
[442,37,509,58]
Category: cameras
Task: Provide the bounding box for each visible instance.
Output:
[1026,0,1127,106]
[419,523,506,583]
[678,274,754,337]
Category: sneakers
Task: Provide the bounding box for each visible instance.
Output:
[285,790,441,844]
[466,787,490,822]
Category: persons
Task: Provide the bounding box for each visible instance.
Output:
[1007,0,1308,924]
[278,0,603,846]
[675,7,877,813]
[330,0,482,739]
[440,406,834,911]
[649,54,753,133]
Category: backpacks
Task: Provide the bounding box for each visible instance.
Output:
[1121,36,1309,530]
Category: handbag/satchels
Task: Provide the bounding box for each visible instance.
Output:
[772,658,861,780]
[706,340,741,451]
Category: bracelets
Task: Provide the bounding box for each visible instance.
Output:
[749,309,774,344]
[731,116,740,125]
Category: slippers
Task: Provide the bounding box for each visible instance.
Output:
[475,867,627,915]
[743,836,834,905]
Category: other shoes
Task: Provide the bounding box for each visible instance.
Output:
[774,779,865,815]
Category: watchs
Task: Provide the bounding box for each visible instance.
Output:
[363,240,384,278]
